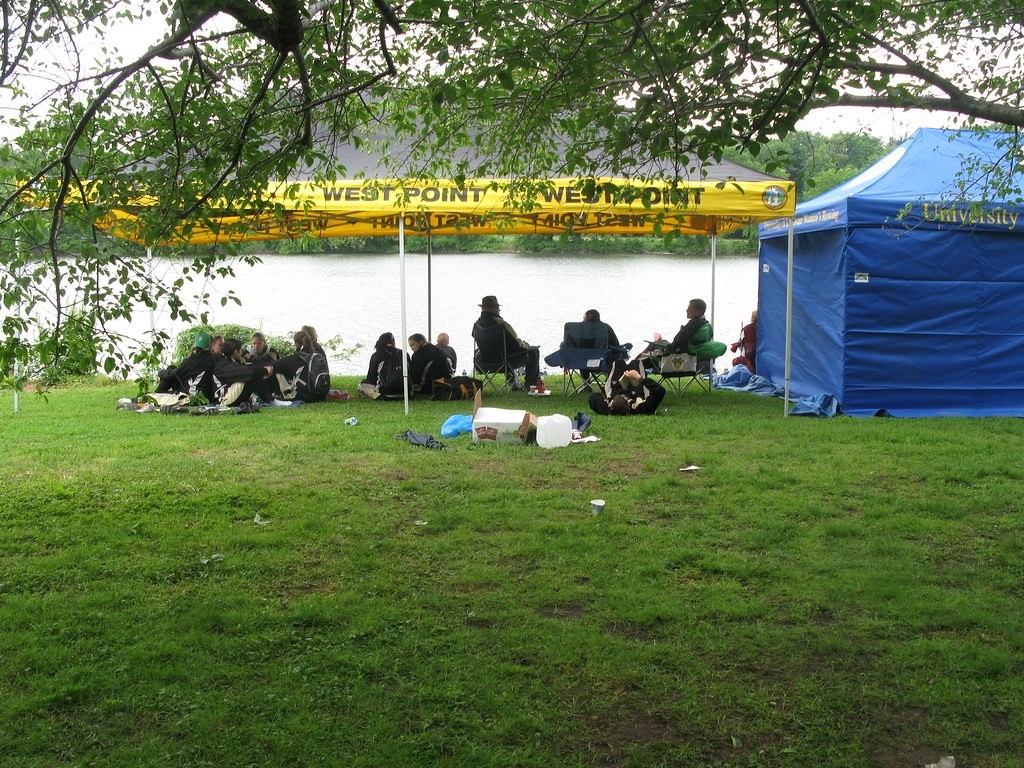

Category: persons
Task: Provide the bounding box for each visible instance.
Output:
[559,309,629,394]
[730,311,758,353]
[361,332,457,402]
[131,325,331,407]
[627,299,713,378]
[472,295,544,391]
[589,370,666,416]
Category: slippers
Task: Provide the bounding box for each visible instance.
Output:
[234,401,260,414]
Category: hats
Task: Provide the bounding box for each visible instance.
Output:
[194,330,215,349]
[478,296,503,306]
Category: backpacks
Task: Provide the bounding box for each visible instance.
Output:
[295,351,331,393]
[378,348,410,395]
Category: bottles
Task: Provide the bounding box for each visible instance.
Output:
[462,369,467,375]
[358,381,361,391]
[536,377,545,393]
[724,368,728,372]
[344,417,357,426]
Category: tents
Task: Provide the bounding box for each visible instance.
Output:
[13,178,796,419]
[713,127,1024,418]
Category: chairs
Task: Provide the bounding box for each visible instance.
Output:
[545,321,633,398]
[644,323,727,399]
[472,322,541,394]
[730,321,757,373]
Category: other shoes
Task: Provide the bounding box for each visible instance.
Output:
[136,402,160,413]
[160,405,189,415]
[127,402,146,411]
[511,381,523,390]
[191,406,219,416]
[326,388,351,400]
[206,403,231,411]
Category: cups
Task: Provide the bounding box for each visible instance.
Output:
[531,386,535,393]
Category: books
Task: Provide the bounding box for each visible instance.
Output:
[618,374,639,390]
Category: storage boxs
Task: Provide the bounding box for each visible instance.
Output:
[470,387,538,444]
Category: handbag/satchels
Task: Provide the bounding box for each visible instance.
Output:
[430,376,483,401]
[441,414,473,438]
[661,347,697,377]
